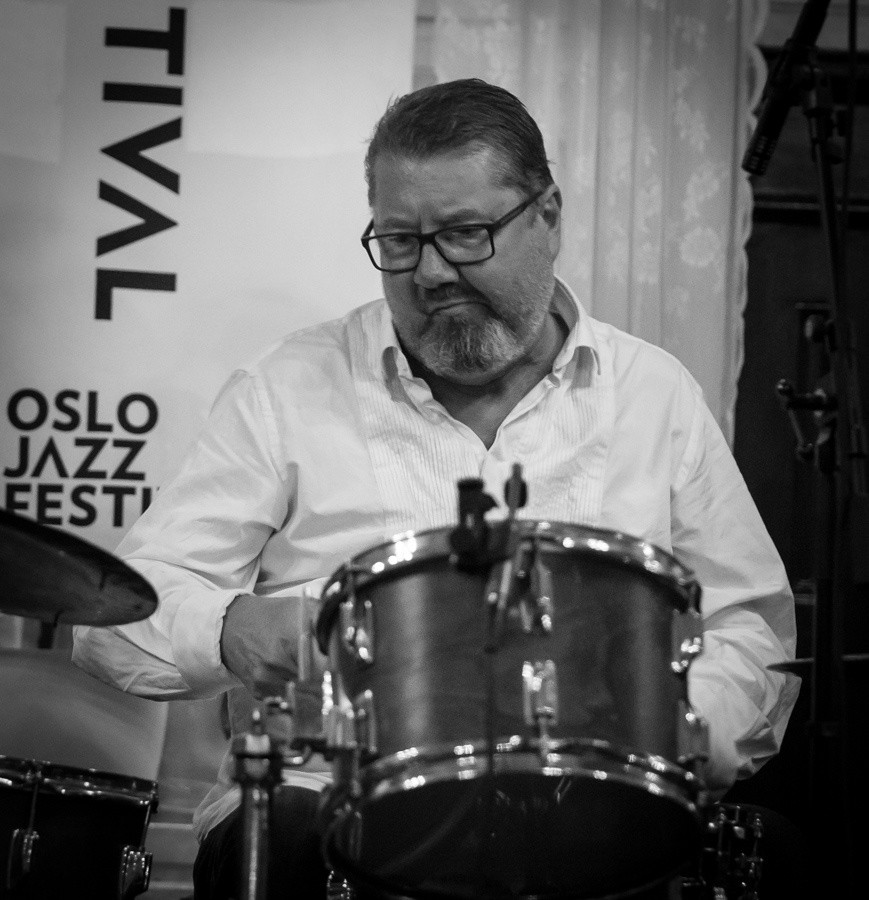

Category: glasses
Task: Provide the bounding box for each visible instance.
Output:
[361,192,540,273]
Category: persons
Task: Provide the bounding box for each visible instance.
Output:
[71,79,802,900]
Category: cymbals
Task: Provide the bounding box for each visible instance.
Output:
[767,653,869,677]
[0,506,159,627]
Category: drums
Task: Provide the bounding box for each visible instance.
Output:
[0,752,160,900]
[315,517,710,900]
